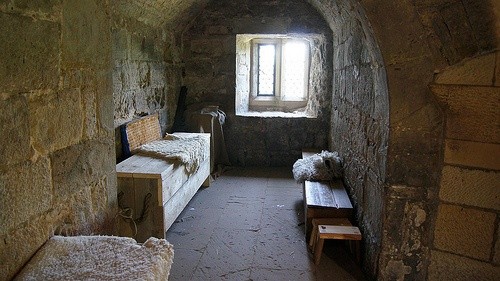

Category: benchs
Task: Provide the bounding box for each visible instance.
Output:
[299,149,355,245]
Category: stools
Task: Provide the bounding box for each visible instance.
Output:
[308,218,362,269]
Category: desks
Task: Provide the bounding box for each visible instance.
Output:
[112,129,212,242]
